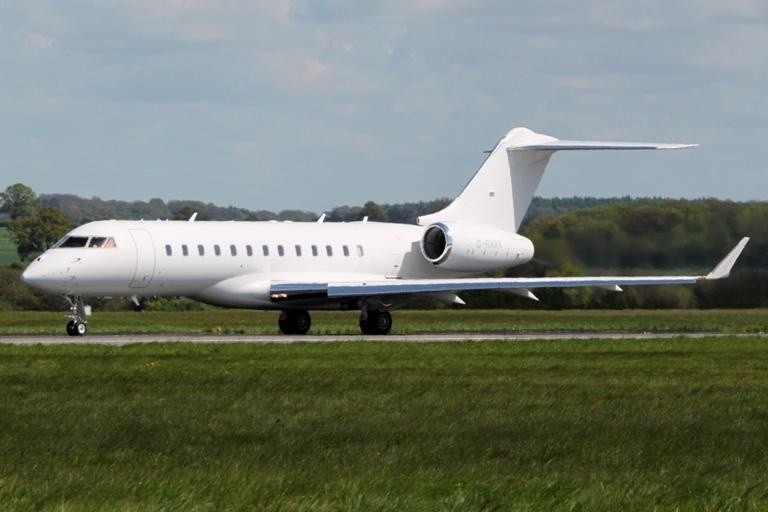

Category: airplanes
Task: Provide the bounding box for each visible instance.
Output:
[20,121,754,336]
